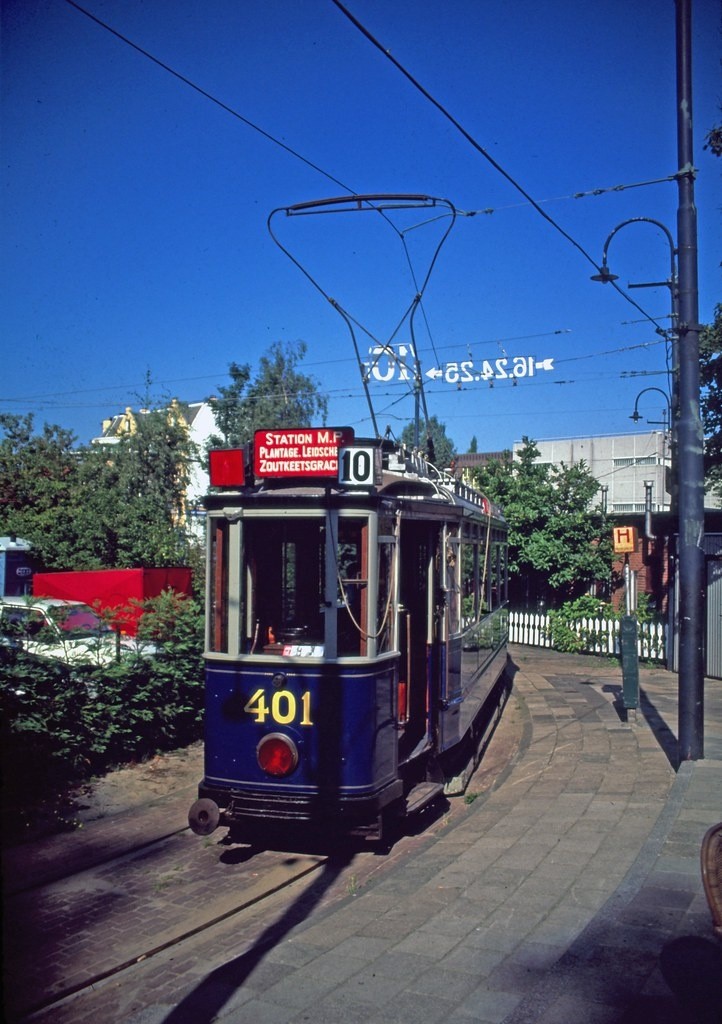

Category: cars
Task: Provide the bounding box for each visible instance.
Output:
[1,595,167,671]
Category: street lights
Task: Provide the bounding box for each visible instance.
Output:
[589,218,708,762]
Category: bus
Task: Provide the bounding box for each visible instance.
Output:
[186,193,510,842]
